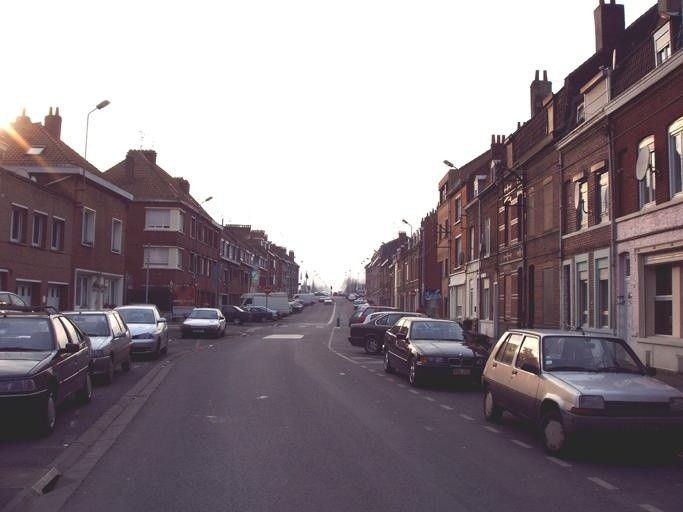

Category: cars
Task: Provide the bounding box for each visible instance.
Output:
[383,316,489,388]
[332,290,399,324]
[58,309,132,383]
[113,304,167,359]
[180,306,226,339]
[481,327,682,460]
[362,312,383,324]
[290,299,303,305]
[287,301,303,312]
[243,306,278,323]
[348,311,423,355]
[314,292,331,304]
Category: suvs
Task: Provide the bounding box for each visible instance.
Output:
[0,306,92,433]
[0,290,30,314]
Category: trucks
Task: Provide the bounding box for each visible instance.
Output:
[288,293,315,305]
[238,292,292,319]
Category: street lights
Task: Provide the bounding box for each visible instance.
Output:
[144,243,151,303]
[401,218,412,239]
[200,194,212,208]
[442,158,460,184]
[490,160,525,328]
[79,97,109,193]
[296,260,327,291]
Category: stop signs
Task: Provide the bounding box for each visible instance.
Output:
[263,287,270,293]
[410,290,416,297]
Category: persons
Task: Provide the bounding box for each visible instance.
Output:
[416,302,426,313]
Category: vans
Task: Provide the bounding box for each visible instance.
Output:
[217,305,249,325]
